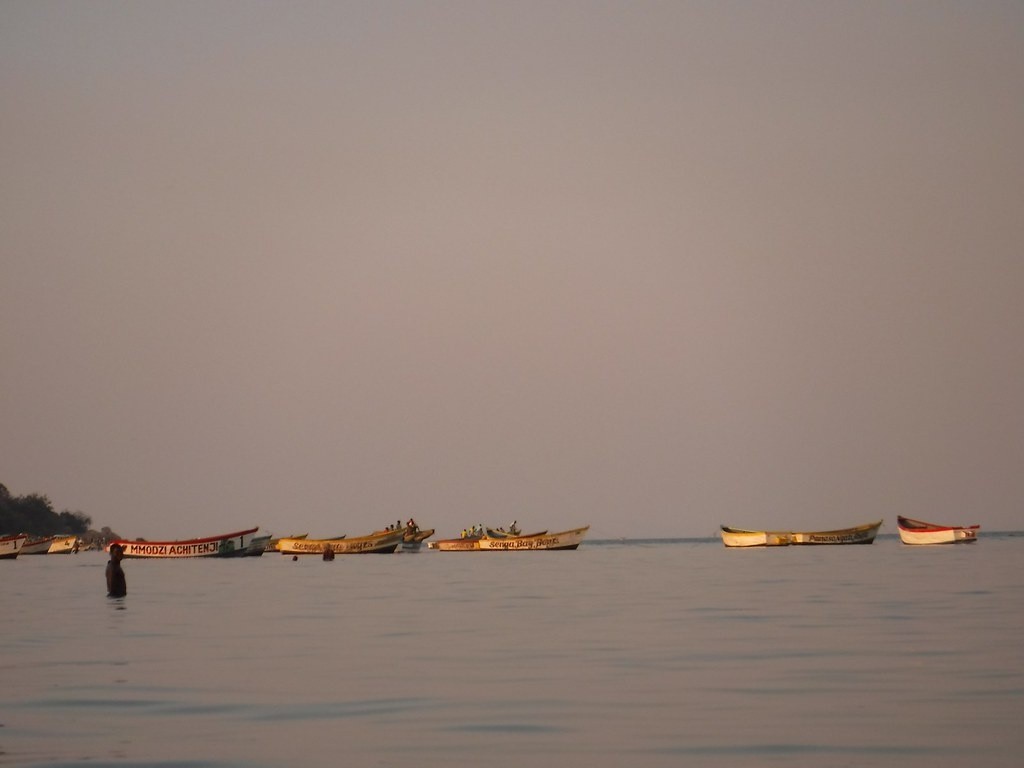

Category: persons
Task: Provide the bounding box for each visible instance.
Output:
[323,543,335,561]
[510,521,517,531]
[461,529,468,538]
[497,527,505,533]
[105,544,126,599]
[407,518,421,534]
[384,525,394,532]
[396,520,402,530]
[469,526,477,537]
[479,524,482,531]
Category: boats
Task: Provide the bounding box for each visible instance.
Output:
[250,529,435,554]
[105,527,272,557]
[427,528,548,552]
[479,524,589,550]
[719,524,791,547]
[896,514,981,544]
[17,538,57,556]
[48,536,79,554]
[0,534,28,560]
[790,518,883,545]
